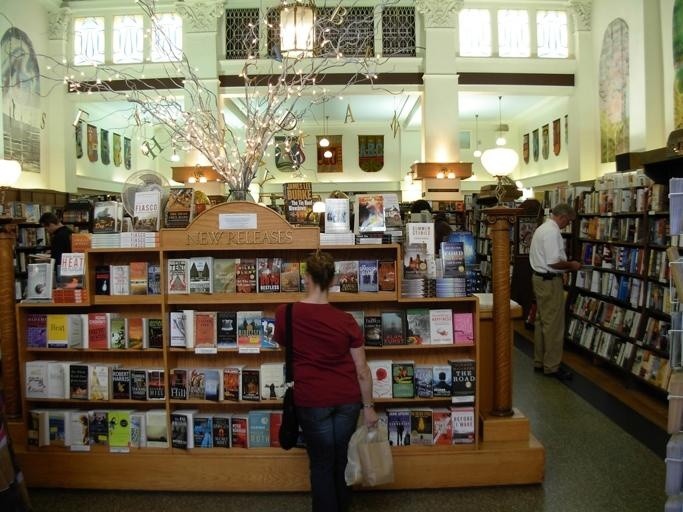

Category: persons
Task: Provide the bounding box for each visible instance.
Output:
[529,204,582,380]
[272,250,381,511]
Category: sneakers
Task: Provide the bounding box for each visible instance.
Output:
[545,368,570,379]
[534,368,543,372]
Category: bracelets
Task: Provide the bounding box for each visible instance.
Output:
[364,402,374,407]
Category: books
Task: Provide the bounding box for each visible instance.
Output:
[27,313,164,349]
[564,178,658,381]
[29,408,169,452]
[170,361,287,401]
[659,177,682,511]
[283,182,474,298]
[542,188,574,234]
[1,187,194,304]
[478,206,491,293]
[168,257,395,295]
[369,359,475,446]
[170,409,305,449]
[170,310,279,349]
[349,310,472,347]
[26,360,165,401]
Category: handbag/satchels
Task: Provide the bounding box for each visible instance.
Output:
[279,387,300,450]
[345,421,388,486]
[357,424,394,488]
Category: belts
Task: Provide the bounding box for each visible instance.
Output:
[535,271,561,277]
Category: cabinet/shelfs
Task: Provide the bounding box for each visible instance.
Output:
[14,247,172,448]
[1,203,63,237]
[158,201,320,451]
[545,186,683,397]
[319,242,545,449]
[441,210,515,292]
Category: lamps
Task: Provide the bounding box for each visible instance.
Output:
[319,97,332,158]
[187,164,207,184]
[279,0,316,59]
[480,148,519,208]
[435,169,456,179]
[495,95,506,146]
[473,113,480,157]
